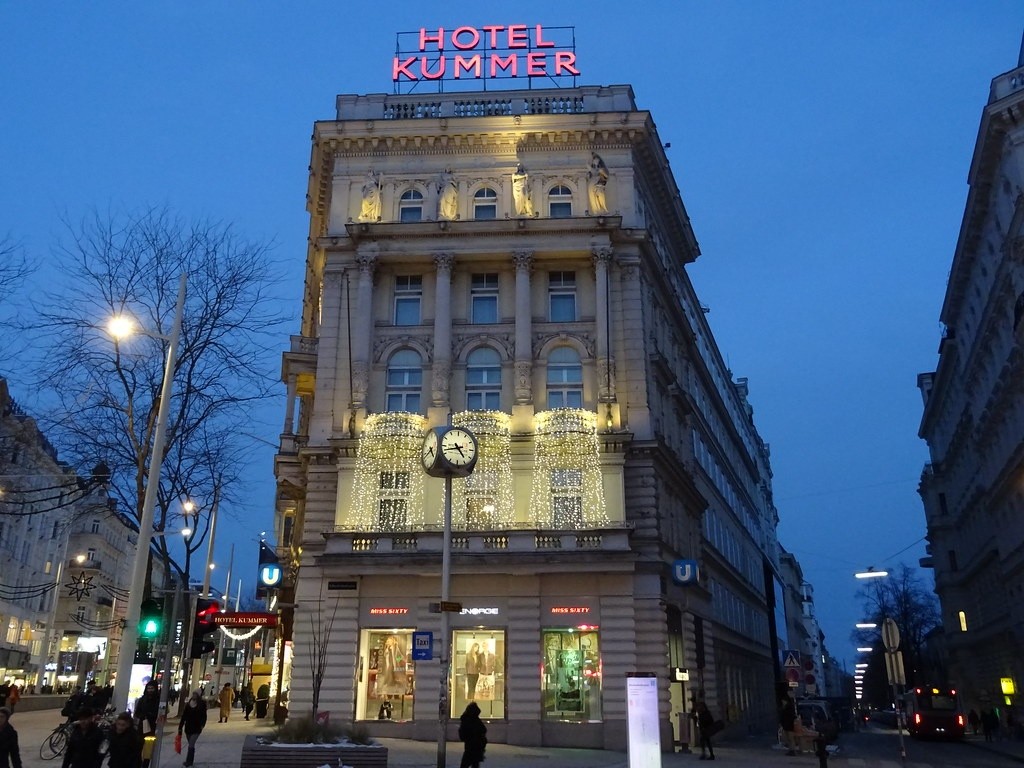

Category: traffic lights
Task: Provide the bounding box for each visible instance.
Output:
[186,598,221,660]
[139,598,162,639]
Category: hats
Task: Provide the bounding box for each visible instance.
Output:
[193,688,202,697]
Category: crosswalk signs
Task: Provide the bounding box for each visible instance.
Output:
[781,649,801,668]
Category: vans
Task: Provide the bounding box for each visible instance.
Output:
[798,700,841,740]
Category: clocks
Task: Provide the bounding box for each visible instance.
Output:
[422,431,437,469]
[442,429,475,466]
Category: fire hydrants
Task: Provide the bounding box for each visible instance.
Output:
[813,732,830,768]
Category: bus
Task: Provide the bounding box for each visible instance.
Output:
[901,684,965,740]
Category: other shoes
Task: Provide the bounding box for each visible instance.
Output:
[707,755,715,760]
[786,751,795,756]
[699,755,705,759]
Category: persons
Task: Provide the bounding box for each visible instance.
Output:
[777,697,796,756]
[512,163,529,215]
[57,680,160,768]
[466,642,496,700]
[357,172,382,220]
[0,707,23,768]
[0,680,20,713]
[178,687,207,768]
[696,699,715,760]
[968,708,1002,743]
[586,154,609,215]
[458,702,488,768]
[383,637,403,686]
[218,683,235,722]
[240,682,255,721]
[439,163,457,221]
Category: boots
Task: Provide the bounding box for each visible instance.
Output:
[183,747,194,766]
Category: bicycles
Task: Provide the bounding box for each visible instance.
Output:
[40,717,73,761]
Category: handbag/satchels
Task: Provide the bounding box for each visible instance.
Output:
[175,736,182,754]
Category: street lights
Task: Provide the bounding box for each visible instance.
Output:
[184,486,220,597]
[105,273,188,720]
[210,543,235,706]
[35,555,85,696]
